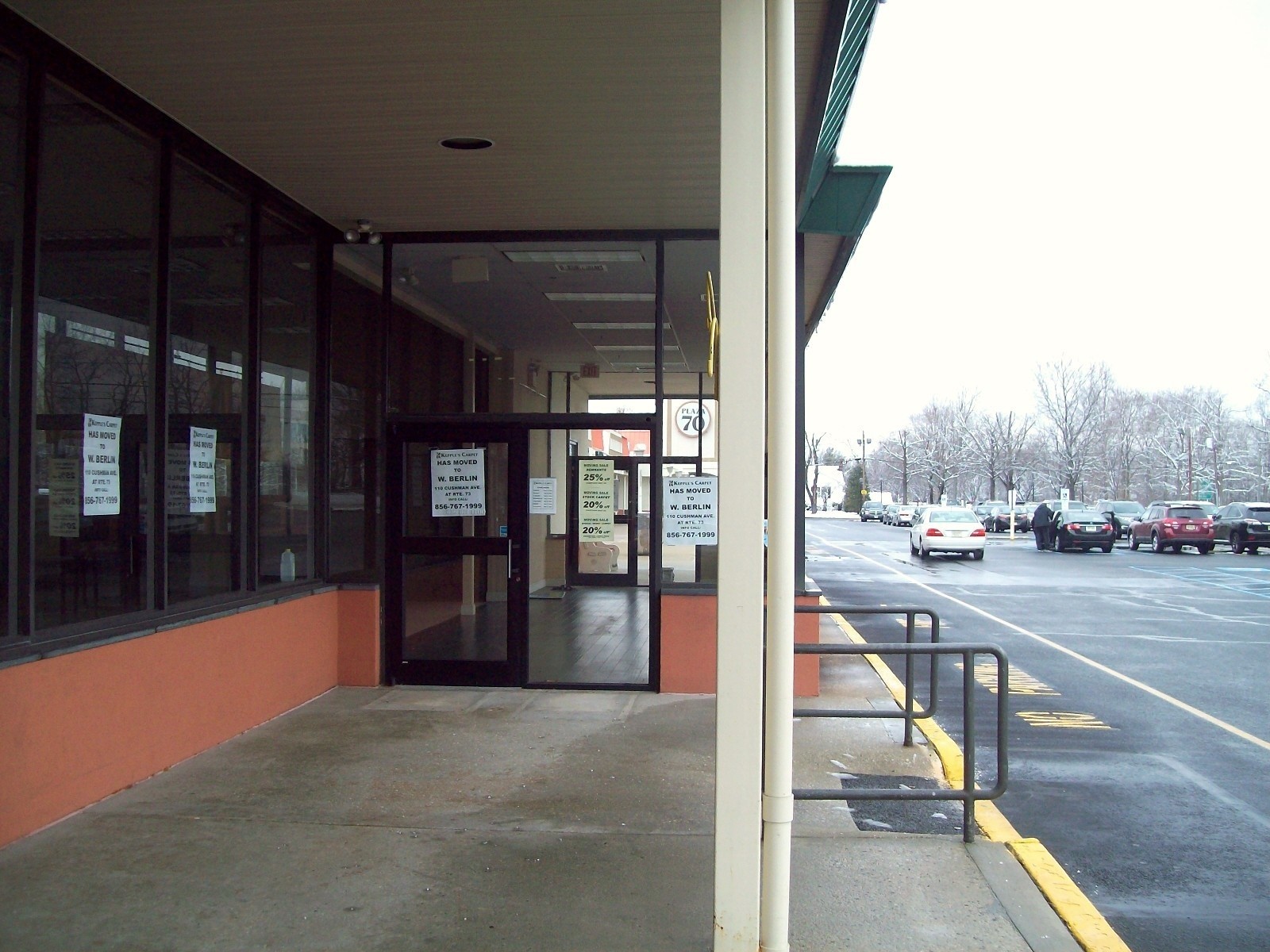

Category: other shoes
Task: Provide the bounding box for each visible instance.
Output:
[1044,549,1052,552]
[1038,549,1043,551]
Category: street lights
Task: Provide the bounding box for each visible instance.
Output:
[1178,425,1193,501]
[855,431,872,507]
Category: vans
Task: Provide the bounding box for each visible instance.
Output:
[860,500,884,523]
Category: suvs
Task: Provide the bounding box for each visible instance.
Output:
[1125,503,1216,556]
[1095,499,1147,540]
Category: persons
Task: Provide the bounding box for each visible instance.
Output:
[1033,503,1054,552]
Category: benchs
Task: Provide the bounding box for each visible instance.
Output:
[934,518,970,522]
[578,542,620,574]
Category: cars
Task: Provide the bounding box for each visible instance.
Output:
[1211,501,1270,554]
[1050,509,1115,554]
[909,504,986,561]
[881,499,1096,532]
[1145,499,1227,521]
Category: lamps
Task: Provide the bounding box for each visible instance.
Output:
[221,224,245,246]
[344,219,382,247]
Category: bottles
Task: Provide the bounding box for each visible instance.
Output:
[280,548,295,582]
